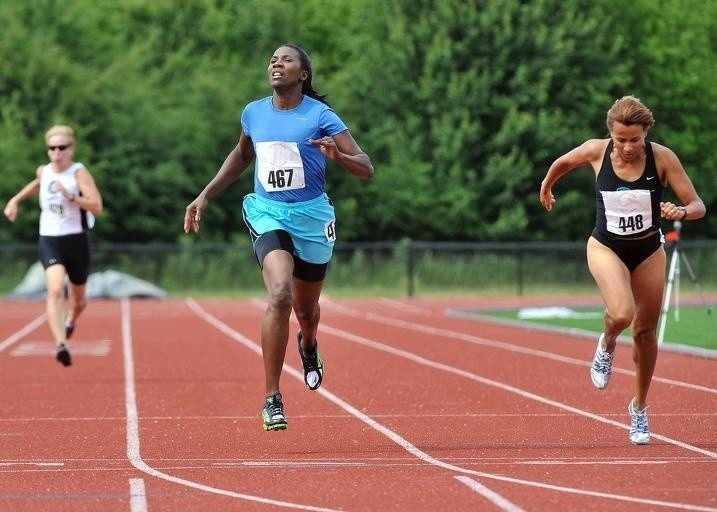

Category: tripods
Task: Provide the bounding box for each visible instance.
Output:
[657,245,711,349]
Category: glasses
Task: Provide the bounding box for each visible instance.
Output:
[45,143,72,151]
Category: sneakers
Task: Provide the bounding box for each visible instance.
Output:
[295,330,324,391]
[590,332,615,390]
[260,392,288,432]
[628,396,650,446]
[63,311,75,339]
[53,343,72,367]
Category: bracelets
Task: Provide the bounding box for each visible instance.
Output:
[680,207,687,221]
[71,194,76,206]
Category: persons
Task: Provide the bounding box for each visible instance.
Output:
[540,95,707,444]
[4,126,103,366]
[184,45,374,431]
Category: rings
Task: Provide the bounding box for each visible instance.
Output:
[670,210,675,215]
[325,138,328,142]
